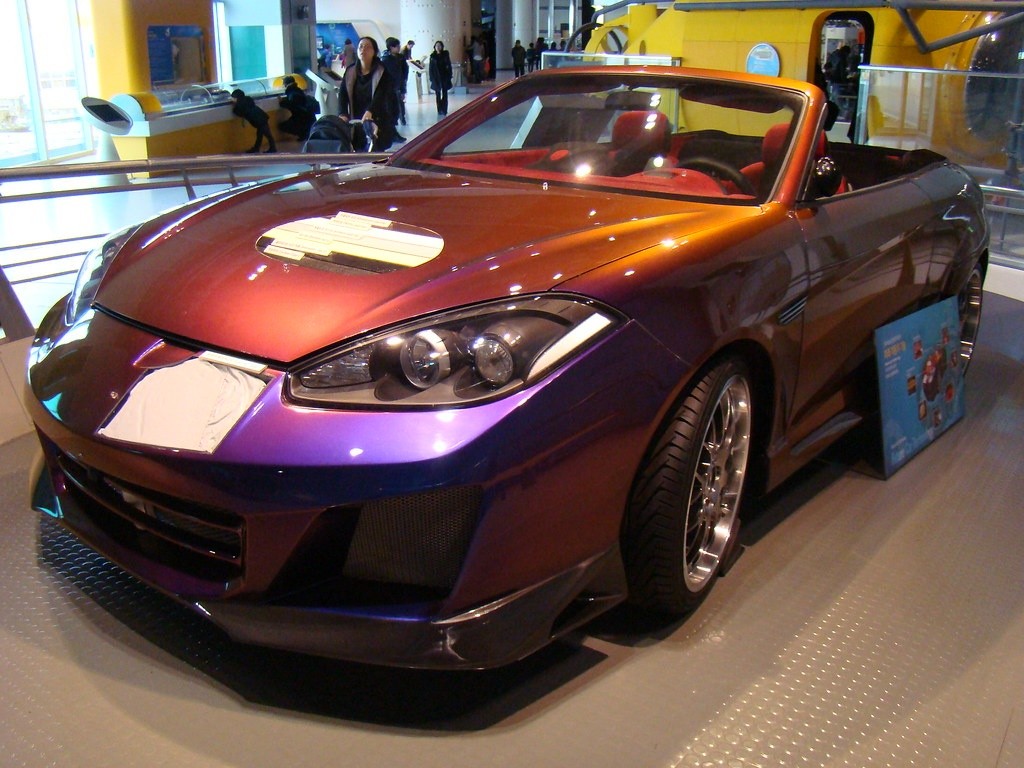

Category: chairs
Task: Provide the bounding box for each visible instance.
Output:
[730,122,850,197]
[599,110,679,176]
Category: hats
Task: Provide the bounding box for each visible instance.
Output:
[231,89,244,99]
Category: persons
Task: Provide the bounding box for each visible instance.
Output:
[466,31,490,83]
[343,39,356,67]
[379,38,408,143]
[338,36,395,153]
[429,41,453,116]
[526,42,536,72]
[320,43,332,68]
[277,76,317,143]
[231,89,278,153]
[551,41,556,50]
[825,39,860,118]
[535,37,549,71]
[512,40,527,78]
[401,40,415,60]
[560,41,566,50]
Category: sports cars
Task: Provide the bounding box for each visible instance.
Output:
[23,62,994,672]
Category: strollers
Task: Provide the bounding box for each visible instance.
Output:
[300,115,377,157]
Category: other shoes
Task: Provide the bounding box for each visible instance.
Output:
[298,136,308,141]
[401,119,406,125]
[439,110,446,115]
[264,148,276,153]
[247,147,259,154]
[392,133,406,142]
